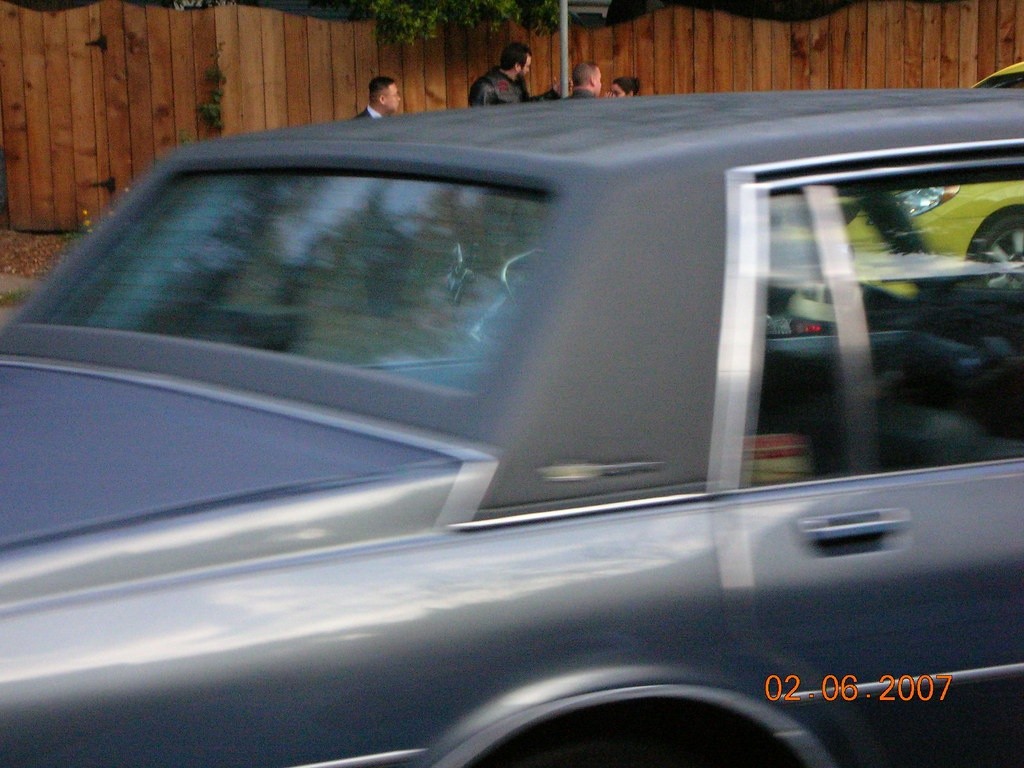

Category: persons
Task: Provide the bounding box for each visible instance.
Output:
[562,61,602,99]
[609,77,640,97]
[353,76,401,120]
[468,40,573,107]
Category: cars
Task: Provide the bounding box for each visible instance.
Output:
[764,59,1024,308]
[0,85,1024,768]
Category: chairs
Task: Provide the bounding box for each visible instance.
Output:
[757,329,992,478]
